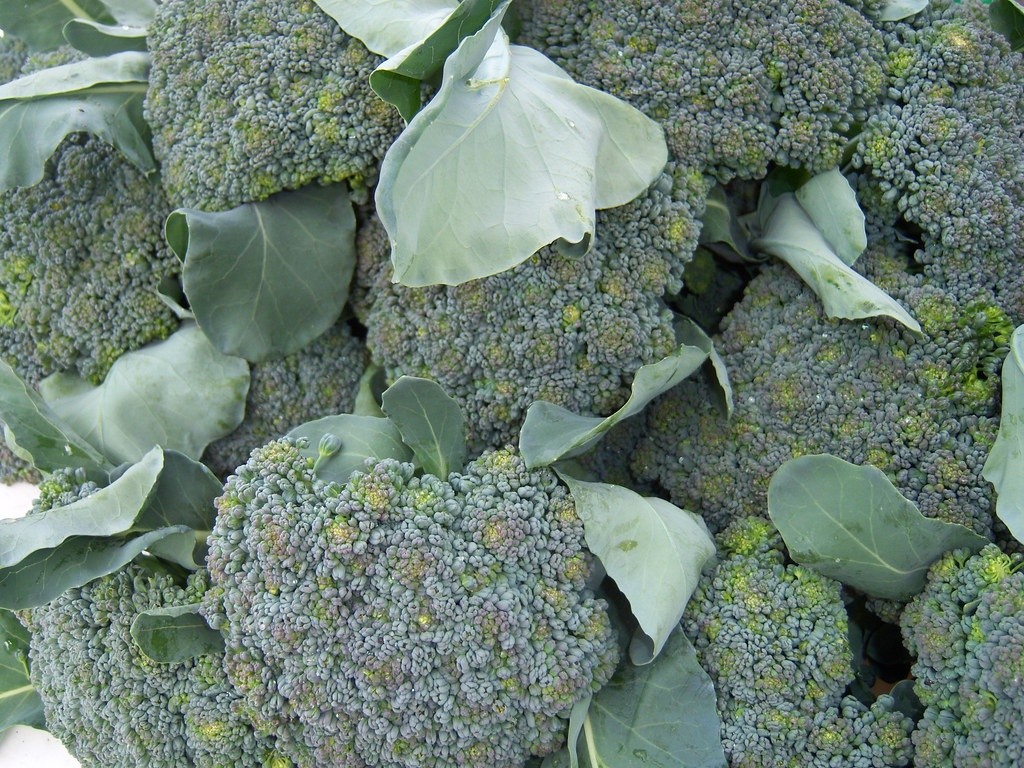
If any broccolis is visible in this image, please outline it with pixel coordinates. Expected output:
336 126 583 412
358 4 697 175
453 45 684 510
0 0 1024 768
